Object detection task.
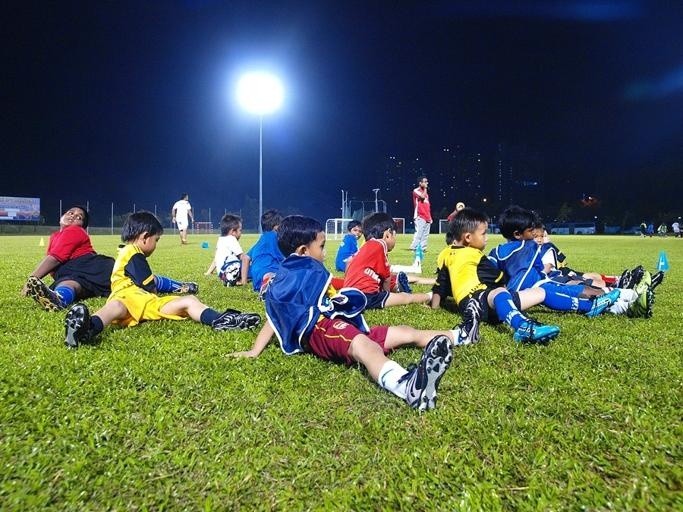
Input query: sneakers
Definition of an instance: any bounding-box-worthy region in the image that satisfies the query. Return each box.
[181,281,199,294]
[629,286,656,319]
[452,298,482,344]
[616,265,663,289]
[64,303,92,347]
[396,270,411,293]
[26,276,64,313]
[413,255,422,273]
[212,309,261,331]
[398,334,453,409]
[513,319,561,344]
[584,288,622,319]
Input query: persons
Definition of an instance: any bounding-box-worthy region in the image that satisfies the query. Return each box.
[448,202,465,221]
[225,215,483,412]
[171,193,195,243]
[204,214,251,287]
[534,214,664,292]
[487,206,653,319]
[335,220,437,284]
[648,221,656,237]
[411,175,433,253]
[421,207,621,346]
[236,209,287,292]
[657,222,668,236]
[544,223,623,283]
[63,211,261,348]
[342,213,434,309]
[432,223,457,274]
[26,206,199,314]
[639,219,647,236]
[672,219,681,237]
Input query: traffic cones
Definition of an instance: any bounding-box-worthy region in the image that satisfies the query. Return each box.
[39,236,45,246]
[656,251,671,272]
